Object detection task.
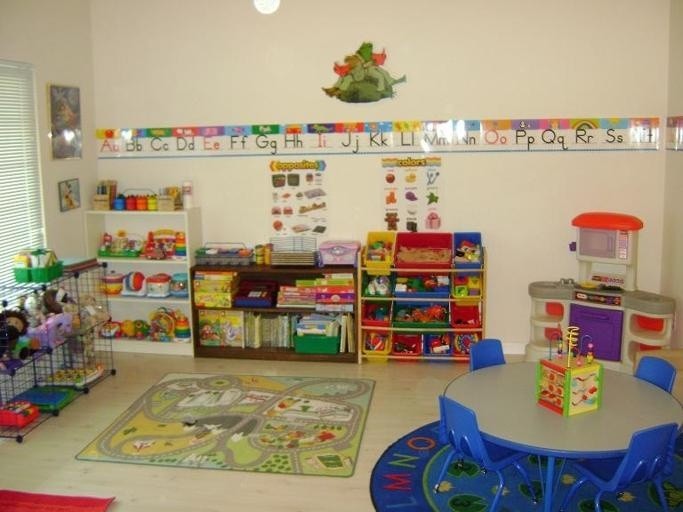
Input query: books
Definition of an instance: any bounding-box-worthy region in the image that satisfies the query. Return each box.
[294,312,340,336]
[243,312,302,349]
[336,312,357,352]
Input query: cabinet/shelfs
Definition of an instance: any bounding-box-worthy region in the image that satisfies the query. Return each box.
[190,263,357,363]
[0,259,117,445]
[83,207,202,357]
[524,279,677,368]
[357,244,488,365]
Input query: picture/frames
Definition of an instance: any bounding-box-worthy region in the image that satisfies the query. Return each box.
[58,177,82,212]
[47,83,84,163]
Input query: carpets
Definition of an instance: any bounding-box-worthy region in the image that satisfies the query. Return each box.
[369,418,682,512]
[74,370,376,478]
[0,489,116,512]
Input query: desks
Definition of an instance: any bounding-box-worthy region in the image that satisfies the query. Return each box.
[443,359,682,512]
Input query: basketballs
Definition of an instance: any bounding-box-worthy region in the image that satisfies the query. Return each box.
[126,271,145,291]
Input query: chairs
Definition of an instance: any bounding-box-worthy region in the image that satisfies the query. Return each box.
[630,354,674,395]
[434,394,537,512]
[560,423,679,512]
[470,338,507,375]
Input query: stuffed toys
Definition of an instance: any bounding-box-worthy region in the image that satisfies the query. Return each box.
[23,290,73,352]
[0,311,39,377]
[40,288,112,329]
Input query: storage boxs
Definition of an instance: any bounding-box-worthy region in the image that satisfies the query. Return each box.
[363,231,397,275]
[392,300,449,336]
[394,276,451,307]
[451,301,483,334]
[361,300,391,335]
[393,232,454,276]
[30,260,64,284]
[10,266,30,284]
[317,241,360,268]
[391,333,422,363]
[454,231,483,277]
[452,277,483,306]
[363,330,392,364]
[452,331,483,365]
[362,271,394,298]
[424,332,453,363]
[292,333,341,357]
